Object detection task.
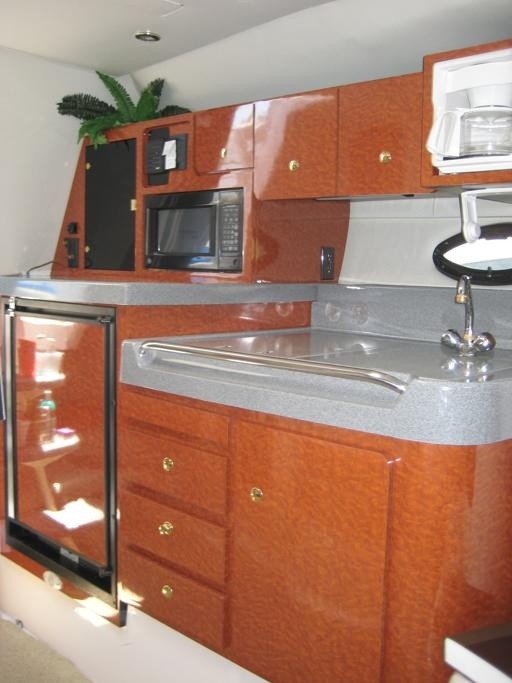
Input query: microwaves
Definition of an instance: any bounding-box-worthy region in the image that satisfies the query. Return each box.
[143,188,245,272]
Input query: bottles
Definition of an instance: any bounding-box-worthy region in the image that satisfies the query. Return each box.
[39,388,57,434]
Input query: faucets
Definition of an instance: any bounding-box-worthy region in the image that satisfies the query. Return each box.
[455,274,474,343]
